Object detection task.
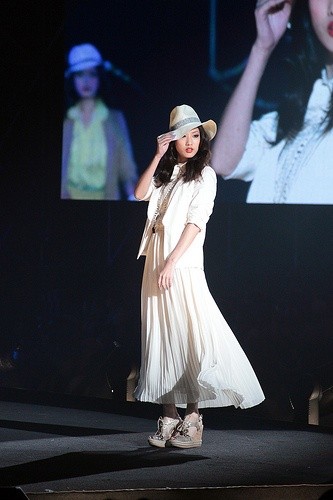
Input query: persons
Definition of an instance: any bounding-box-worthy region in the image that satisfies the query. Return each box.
[130,105,265,448]
[210,0,333,204]
[59,43,139,201]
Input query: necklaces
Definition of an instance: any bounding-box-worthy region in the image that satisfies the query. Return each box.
[153,173,182,220]
[272,124,317,202]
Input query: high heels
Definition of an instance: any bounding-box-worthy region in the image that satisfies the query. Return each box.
[147,417,182,448]
[167,414,204,448]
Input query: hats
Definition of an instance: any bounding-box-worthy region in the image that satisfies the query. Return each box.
[154,104,219,143]
